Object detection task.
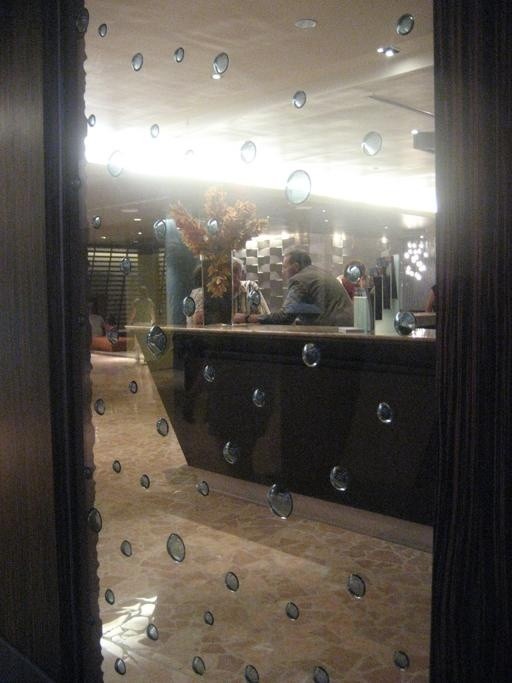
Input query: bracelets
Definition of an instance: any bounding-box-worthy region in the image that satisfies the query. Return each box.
[243,313,251,324]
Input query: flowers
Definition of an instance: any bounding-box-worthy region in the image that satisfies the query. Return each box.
[167,185,270,300]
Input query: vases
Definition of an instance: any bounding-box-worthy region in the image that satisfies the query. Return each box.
[201,248,235,327]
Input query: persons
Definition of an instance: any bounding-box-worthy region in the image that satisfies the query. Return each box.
[233,259,271,313]
[128,285,155,326]
[231,250,357,325]
[425,283,436,315]
[186,265,206,327]
[335,259,369,297]
